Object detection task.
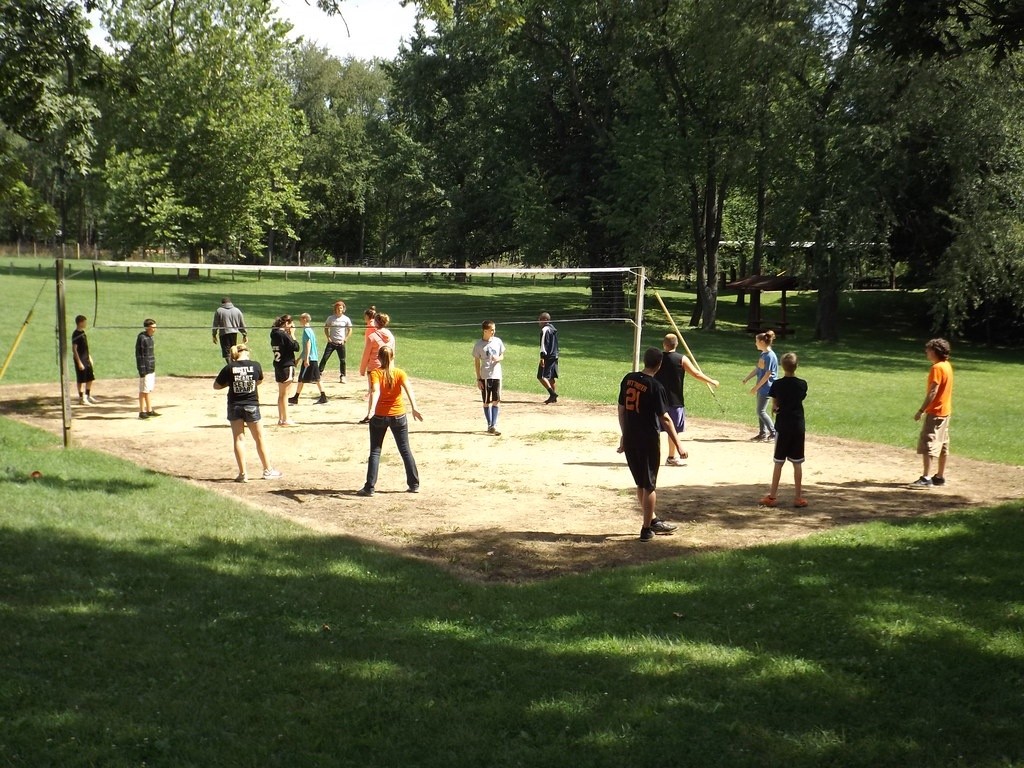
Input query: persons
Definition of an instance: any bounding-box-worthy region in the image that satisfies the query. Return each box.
[537,312,559,404]
[359,313,395,424]
[289,313,327,404]
[212,298,247,364]
[72,315,99,405]
[136,319,162,419]
[355,346,424,497]
[364,306,377,343]
[270,314,300,424]
[742,330,777,443]
[318,301,352,382]
[653,334,719,467]
[909,337,953,486]
[758,352,809,507]
[616,346,688,542]
[214,344,282,482]
[472,320,505,435]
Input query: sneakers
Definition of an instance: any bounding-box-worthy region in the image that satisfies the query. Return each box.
[263,468,283,479]
[907,477,933,489]
[931,473,945,486]
[764,432,777,442]
[758,496,776,506]
[650,519,679,534]
[665,457,688,467]
[794,498,809,507]
[357,488,374,497]
[749,432,766,443]
[640,527,656,541]
[409,483,419,493]
[359,417,371,423]
[235,474,248,483]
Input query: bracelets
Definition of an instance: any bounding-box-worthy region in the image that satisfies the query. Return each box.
[919,409,924,413]
[477,379,481,381]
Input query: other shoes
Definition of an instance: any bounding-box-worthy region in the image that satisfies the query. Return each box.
[79,398,92,406]
[139,412,150,420]
[340,376,347,383]
[147,411,162,416]
[544,393,558,404]
[488,425,502,435]
[288,397,298,404]
[313,400,329,404]
[87,397,100,404]
[278,418,301,427]
